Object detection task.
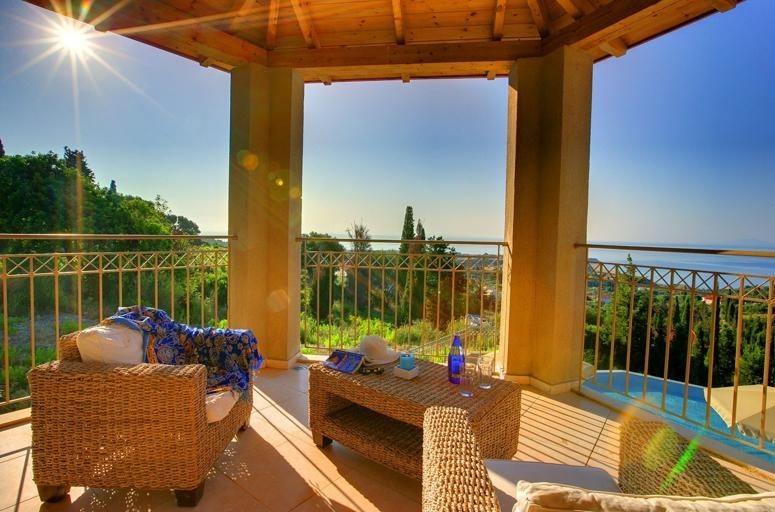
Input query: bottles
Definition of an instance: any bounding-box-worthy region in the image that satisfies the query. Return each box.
[448,331,465,384]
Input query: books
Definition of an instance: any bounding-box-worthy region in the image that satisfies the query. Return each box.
[323,349,376,375]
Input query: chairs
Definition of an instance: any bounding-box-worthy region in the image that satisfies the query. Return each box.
[27,302,258,505]
[421,393,775,511]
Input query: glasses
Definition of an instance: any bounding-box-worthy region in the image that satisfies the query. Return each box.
[358,366,384,375]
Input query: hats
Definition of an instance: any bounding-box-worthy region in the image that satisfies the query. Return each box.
[349,335,401,366]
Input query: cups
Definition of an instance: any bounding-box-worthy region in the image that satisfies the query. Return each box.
[459,356,493,398]
